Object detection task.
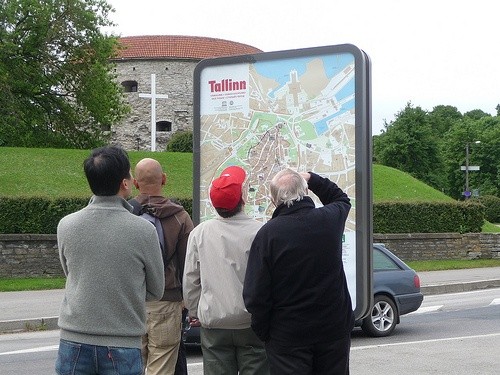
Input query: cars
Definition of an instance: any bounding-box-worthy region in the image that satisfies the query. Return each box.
[182,243,424,347]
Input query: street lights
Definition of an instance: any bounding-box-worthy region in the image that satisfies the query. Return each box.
[466,140,481,199]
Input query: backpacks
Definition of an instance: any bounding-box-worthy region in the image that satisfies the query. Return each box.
[138,212,166,264]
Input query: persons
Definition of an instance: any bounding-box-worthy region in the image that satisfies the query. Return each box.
[128,158,196,375]
[55,147,165,375]
[243,168,356,375]
[182,166,268,375]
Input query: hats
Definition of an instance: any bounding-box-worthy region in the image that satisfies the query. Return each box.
[210,166,246,211]
